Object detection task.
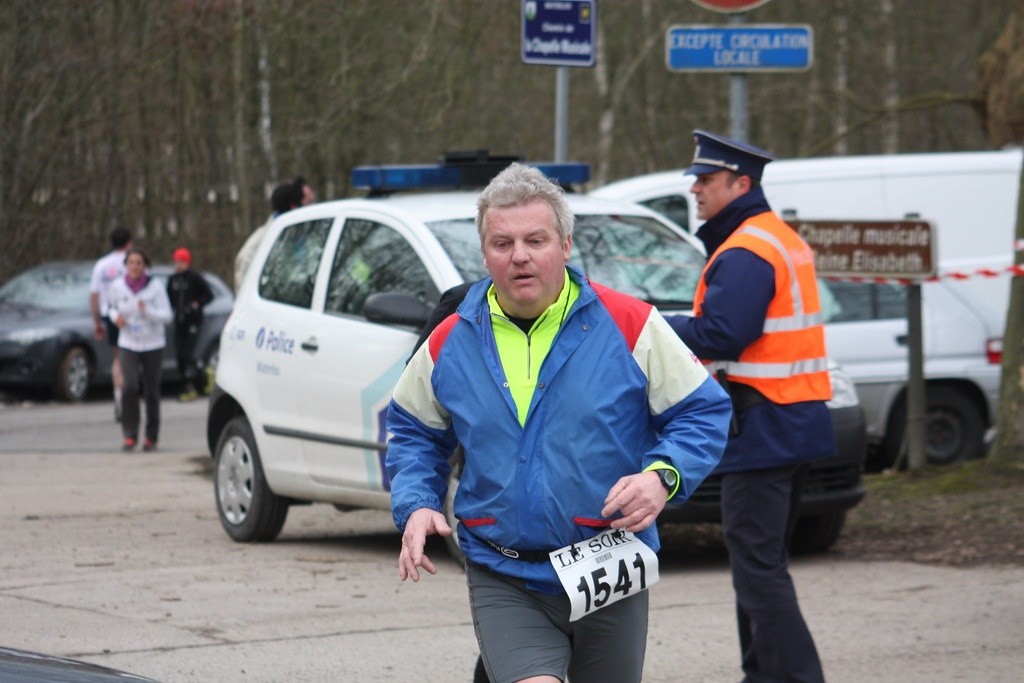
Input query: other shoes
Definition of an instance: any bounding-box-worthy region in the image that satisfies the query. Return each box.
[203,367,214,394]
[123,437,137,450]
[179,389,198,401]
[143,438,153,450]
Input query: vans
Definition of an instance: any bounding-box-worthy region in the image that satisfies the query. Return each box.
[581,147,1024,471]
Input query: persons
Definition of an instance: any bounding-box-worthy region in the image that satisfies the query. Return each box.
[380,130,837,683]
[90,224,215,452]
[271,176,316,217]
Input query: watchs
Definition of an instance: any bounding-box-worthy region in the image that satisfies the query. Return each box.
[654,469,677,495]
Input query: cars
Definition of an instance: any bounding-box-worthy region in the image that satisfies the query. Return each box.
[204,147,868,559]
[1,255,234,402]
[819,272,996,466]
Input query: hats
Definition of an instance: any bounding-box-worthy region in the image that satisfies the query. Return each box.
[683,128,774,181]
[173,248,192,264]
